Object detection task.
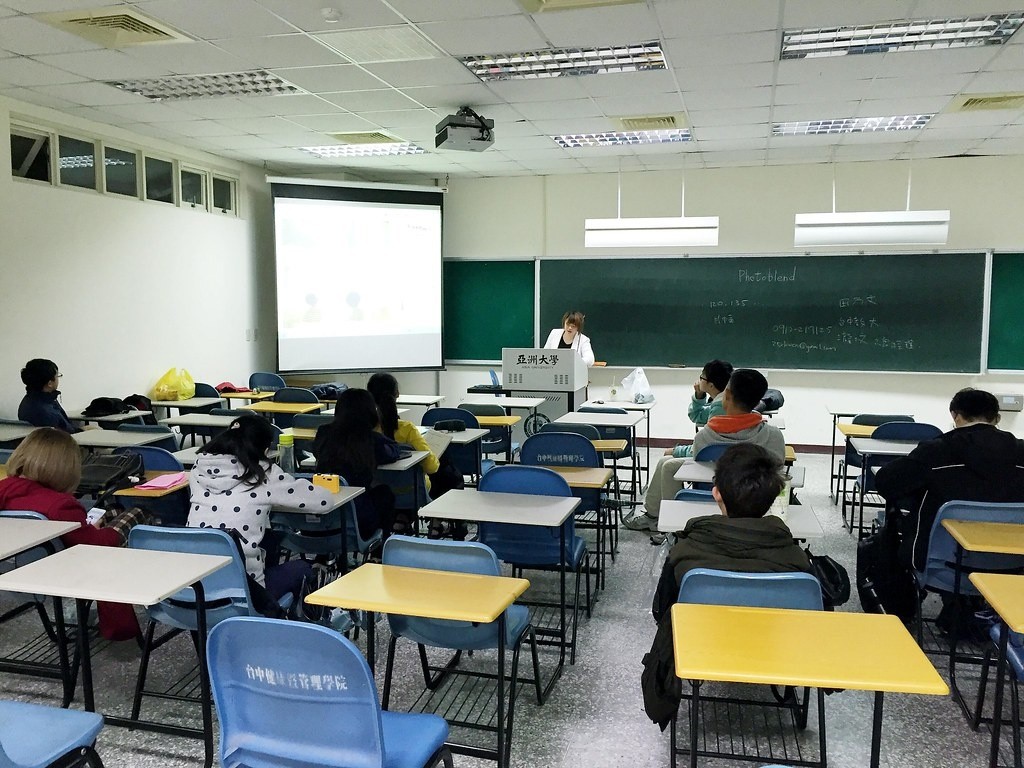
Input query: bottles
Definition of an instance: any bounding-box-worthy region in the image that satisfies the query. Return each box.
[277,434,294,477]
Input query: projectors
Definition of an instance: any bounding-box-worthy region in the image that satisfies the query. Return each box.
[434,126,495,152]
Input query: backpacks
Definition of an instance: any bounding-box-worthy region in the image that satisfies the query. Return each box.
[853,511,923,624]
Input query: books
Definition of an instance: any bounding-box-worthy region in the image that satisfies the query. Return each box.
[424,429,453,459]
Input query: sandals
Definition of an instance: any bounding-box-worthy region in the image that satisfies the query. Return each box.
[427,524,450,540]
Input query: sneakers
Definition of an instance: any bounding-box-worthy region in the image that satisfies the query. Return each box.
[625,510,658,531]
[650,533,668,545]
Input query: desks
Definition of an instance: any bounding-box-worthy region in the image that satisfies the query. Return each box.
[0,392,1024,768]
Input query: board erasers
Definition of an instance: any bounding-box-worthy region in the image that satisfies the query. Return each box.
[593,361,607,367]
[669,364,685,368]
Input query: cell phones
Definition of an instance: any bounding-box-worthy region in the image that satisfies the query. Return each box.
[86,507,106,524]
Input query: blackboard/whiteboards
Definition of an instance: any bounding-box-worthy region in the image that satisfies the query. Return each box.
[538,248,989,375]
[986,250,1024,374]
[442,256,537,364]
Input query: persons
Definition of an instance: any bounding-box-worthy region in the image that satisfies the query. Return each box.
[693,369,785,467]
[544,312,594,367]
[641,442,811,732]
[17,358,113,459]
[367,372,452,537]
[0,427,157,639]
[877,388,1024,647]
[313,389,400,563]
[624,359,734,544]
[186,415,333,629]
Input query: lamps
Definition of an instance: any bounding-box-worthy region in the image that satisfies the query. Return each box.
[583,152,720,248]
[792,139,951,247]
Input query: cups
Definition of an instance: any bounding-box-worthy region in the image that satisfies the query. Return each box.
[771,474,793,521]
[608,386,618,402]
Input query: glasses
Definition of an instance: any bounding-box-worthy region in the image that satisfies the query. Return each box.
[53,373,63,379]
[699,374,708,382]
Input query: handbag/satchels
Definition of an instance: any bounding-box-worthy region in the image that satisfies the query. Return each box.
[310,381,348,399]
[148,367,195,401]
[621,368,655,404]
[285,553,339,624]
[84,397,129,416]
[76,450,145,491]
[761,390,784,410]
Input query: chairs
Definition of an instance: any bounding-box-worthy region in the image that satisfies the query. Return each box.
[117,423,180,453]
[0,698,106,768]
[457,403,521,465]
[380,533,543,768]
[692,442,734,491]
[0,509,100,644]
[376,443,435,510]
[249,372,287,424]
[669,566,825,768]
[989,622,1024,768]
[210,408,257,417]
[476,464,591,667]
[673,488,716,501]
[178,383,223,450]
[834,413,916,529]
[203,616,455,768]
[293,413,335,473]
[0,448,15,465]
[127,404,145,425]
[0,418,34,427]
[906,499,1024,658]
[850,420,944,542]
[421,407,495,488]
[539,422,627,554]
[127,524,294,733]
[577,406,643,510]
[520,432,615,574]
[110,446,191,524]
[273,387,322,469]
